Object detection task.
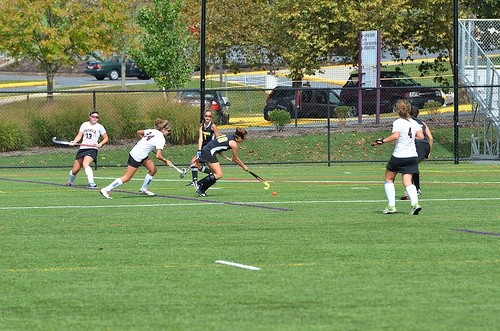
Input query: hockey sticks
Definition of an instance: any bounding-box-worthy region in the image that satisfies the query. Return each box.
[52,137,102,148]
[151,149,186,174]
[180,156,199,179]
[219,153,270,190]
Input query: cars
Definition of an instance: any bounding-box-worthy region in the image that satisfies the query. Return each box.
[83,54,151,80]
[177,88,231,125]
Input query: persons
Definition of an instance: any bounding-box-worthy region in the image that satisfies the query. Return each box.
[303,82,311,86]
[99,119,171,199]
[401,107,433,200]
[187,110,220,186]
[371,100,425,215]
[66,112,108,187]
[192,127,250,197]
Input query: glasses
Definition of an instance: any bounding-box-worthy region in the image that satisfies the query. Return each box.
[91,116,99,119]
[166,129,171,132]
[204,115,212,118]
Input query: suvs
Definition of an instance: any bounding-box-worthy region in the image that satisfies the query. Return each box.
[263,86,341,121]
[340,71,445,117]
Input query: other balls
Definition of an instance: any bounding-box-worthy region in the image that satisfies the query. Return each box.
[272,192,278,196]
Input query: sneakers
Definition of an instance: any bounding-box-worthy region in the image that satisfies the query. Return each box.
[139,188,155,196]
[383,206,397,214]
[100,187,113,199]
[193,180,199,191]
[409,203,422,216]
[88,183,97,187]
[401,189,421,200]
[196,190,206,197]
[65,182,72,186]
